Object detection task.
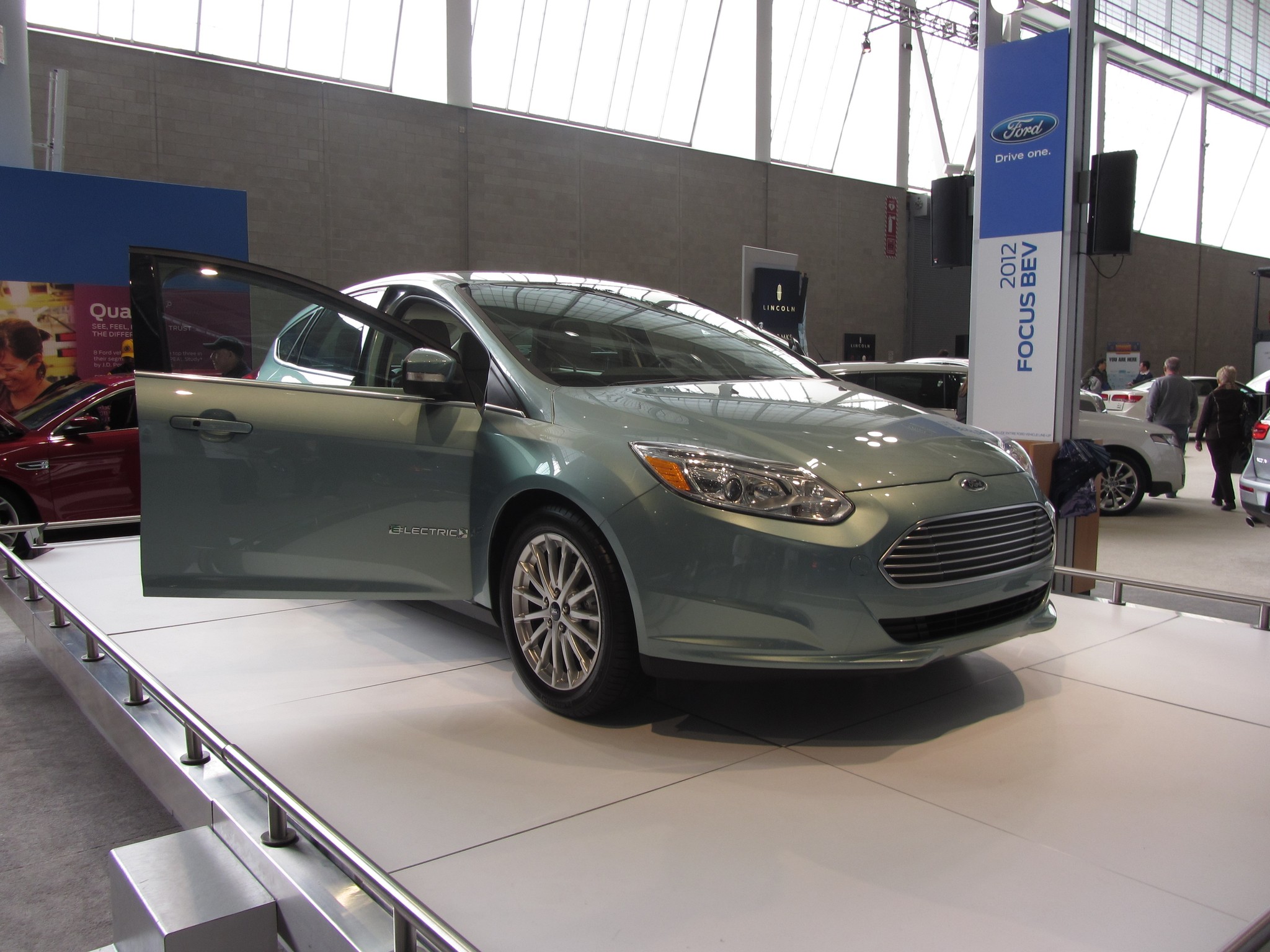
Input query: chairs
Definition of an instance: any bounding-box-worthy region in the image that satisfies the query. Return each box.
[537,319,595,371]
[109,399,138,429]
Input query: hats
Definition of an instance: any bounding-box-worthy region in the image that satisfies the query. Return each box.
[121,338,134,359]
[203,336,245,357]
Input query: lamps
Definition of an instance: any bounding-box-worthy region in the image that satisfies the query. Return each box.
[968,12,978,22]
[861,34,871,54]
[902,42,912,51]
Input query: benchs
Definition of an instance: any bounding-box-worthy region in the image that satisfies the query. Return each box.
[388,320,490,406]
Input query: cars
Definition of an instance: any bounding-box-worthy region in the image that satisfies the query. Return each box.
[2,370,223,557]
[129,234,1056,715]
[819,357,1269,529]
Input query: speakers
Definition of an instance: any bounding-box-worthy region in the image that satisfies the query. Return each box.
[1086,150,1139,256]
[931,174,975,270]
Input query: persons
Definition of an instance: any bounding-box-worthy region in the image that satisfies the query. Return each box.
[1194,365,1245,510]
[1080,359,1112,395]
[0,318,64,412]
[104,337,138,430]
[937,349,949,357]
[1125,361,1153,386]
[201,336,252,378]
[64,375,101,434]
[1146,357,1199,497]
